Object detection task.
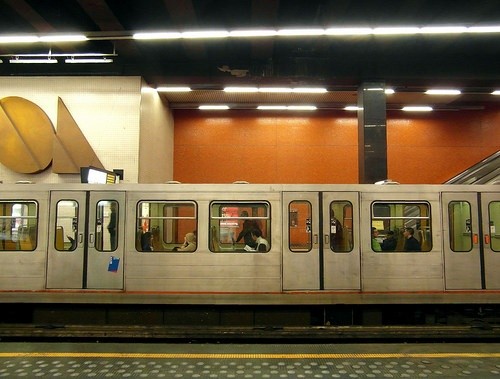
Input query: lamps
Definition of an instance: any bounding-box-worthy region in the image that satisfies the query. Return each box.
[0,48,117,64]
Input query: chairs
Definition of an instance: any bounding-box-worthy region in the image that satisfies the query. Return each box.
[395,228,428,251]
[137,225,164,251]
[211,225,220,251]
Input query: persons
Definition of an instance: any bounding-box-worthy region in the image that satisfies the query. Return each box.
[235,210,262,251]
[330,208,343,253]
[371,227,383,252]
[248,228,270,253]
[140,230,154,252]
[106,201,117,251]
[379,230,398,252]
[403,226,421,252]
[177,232,197,253]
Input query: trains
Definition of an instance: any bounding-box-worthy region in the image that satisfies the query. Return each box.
[0,178,500,343]
